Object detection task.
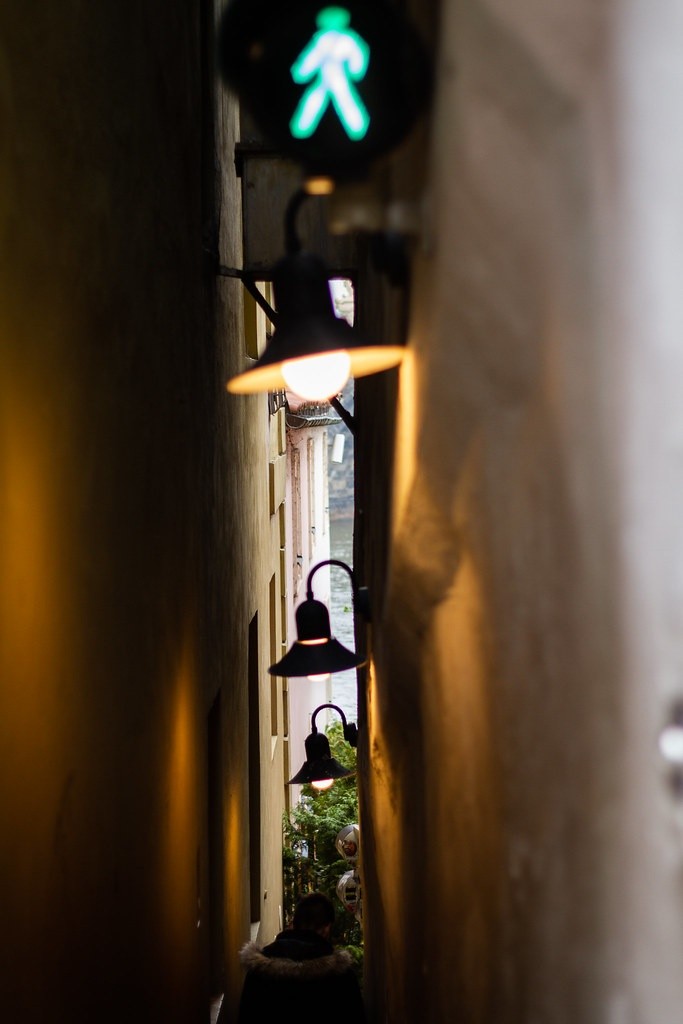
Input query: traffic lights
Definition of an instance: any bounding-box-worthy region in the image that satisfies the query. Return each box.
[217,0,439,171]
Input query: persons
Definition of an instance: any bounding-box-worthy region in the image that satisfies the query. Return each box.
[237,892,367,1024]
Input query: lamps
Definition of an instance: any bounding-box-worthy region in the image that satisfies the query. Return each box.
[267,560,373,678]
[287,703,358,789]
[225,160,405,404]
